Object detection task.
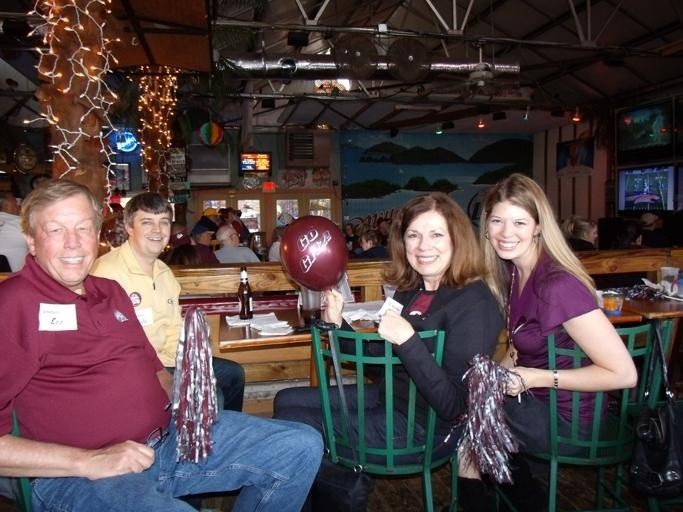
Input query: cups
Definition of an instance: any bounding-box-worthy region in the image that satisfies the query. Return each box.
[661,266,679,298]
[302,286,321,327]
[602,293,624,315]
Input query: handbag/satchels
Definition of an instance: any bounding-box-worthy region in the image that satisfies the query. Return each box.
[626,318,682,499]
[309,460,369,512]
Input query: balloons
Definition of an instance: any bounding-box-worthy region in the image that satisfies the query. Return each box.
[279,217,347,292]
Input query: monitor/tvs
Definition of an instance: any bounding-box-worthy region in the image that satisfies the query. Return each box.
[615,160,683,214]
[239,152,272,176]
[615,95,679,163]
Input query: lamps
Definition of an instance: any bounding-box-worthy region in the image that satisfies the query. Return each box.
[433,106,581,135]
[389,108,506,138]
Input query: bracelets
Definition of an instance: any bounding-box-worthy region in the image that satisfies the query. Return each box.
[552,368,559,392]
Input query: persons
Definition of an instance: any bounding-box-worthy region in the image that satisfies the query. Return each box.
[456,173,640,510]
[616,217,649,252]
[560,212,598,250]
[270,188,509,511]
[169,204,391,270]
[639,212,670,248]
[85,193,244,416]
[0,175,325,511]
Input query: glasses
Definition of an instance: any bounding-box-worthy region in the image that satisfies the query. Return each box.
[146,427,170,452]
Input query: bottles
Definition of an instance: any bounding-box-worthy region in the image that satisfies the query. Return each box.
[238,265,254,319]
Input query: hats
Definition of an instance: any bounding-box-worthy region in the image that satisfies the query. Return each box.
[191,225,214,234]
[639,212,659,226]
[203,207,221,217]
